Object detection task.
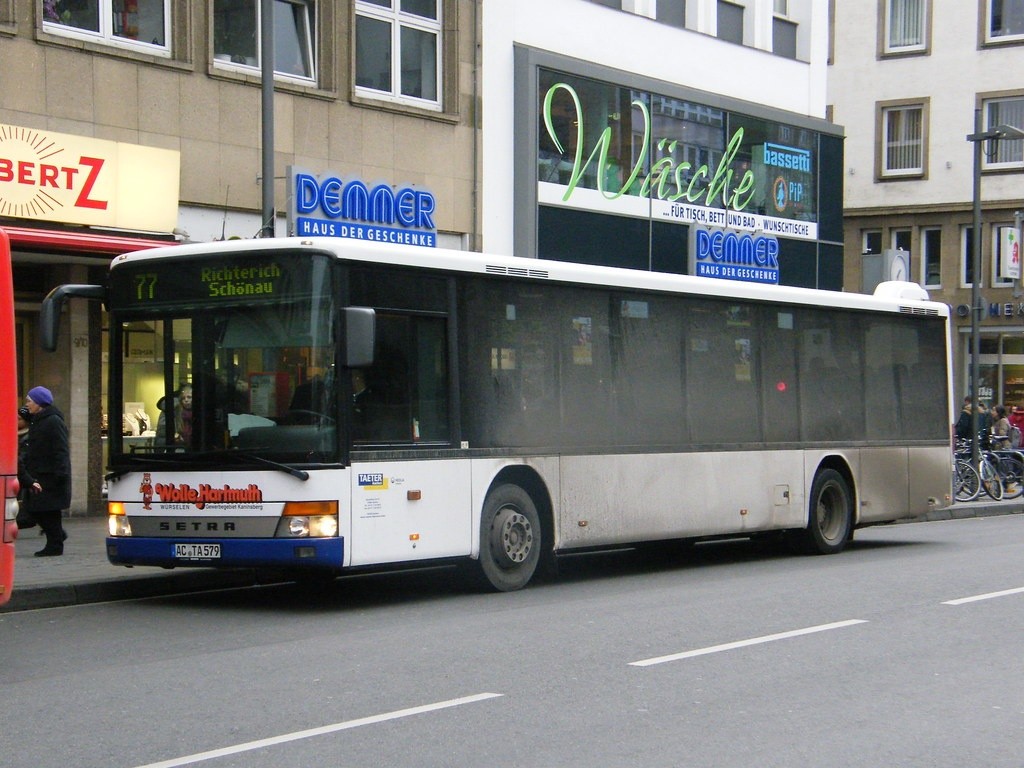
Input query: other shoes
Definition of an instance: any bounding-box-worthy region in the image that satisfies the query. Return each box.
[34,532,69,556]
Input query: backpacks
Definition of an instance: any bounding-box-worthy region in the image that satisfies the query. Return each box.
[1004,423,1022,449]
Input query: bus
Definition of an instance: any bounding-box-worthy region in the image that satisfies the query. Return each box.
[40,237,953,592]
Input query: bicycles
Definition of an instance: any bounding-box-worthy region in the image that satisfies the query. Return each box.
[953,435,1024,502]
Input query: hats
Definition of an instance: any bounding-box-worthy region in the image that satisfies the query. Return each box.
[28,386,53,407]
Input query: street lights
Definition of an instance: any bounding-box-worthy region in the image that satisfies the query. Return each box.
[966,125,1024,501]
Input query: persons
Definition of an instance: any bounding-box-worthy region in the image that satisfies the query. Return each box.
[16,406,33,529]
[953,390,1024,493]
[152,372,231,456]
[25,386,73,558]
